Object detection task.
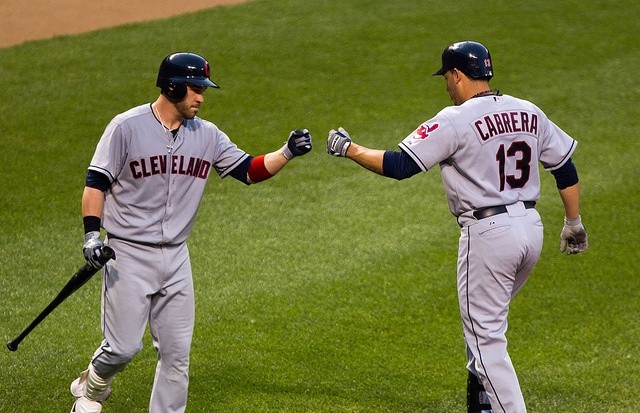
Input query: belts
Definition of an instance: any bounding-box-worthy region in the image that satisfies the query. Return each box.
[107,231,122,242]
[457,200,537,229]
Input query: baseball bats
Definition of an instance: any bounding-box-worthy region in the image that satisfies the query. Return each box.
[6,246,114,352]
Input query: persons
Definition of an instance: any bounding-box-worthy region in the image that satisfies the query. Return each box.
[68,52,314,413]
[325,41,589,413]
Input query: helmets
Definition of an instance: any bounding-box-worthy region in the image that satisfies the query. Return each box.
[156,52,220,102]
[433,40,494,82]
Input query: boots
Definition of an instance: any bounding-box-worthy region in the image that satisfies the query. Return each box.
[69,359,114,413]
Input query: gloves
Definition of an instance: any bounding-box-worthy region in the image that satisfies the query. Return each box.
[326,126,351,157]
[83,229,104,270]
[559,216,588,256]
[282,129,312,160]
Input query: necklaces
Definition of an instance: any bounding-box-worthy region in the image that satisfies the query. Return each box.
[469,89,500,98]
[152,103,184,154]
